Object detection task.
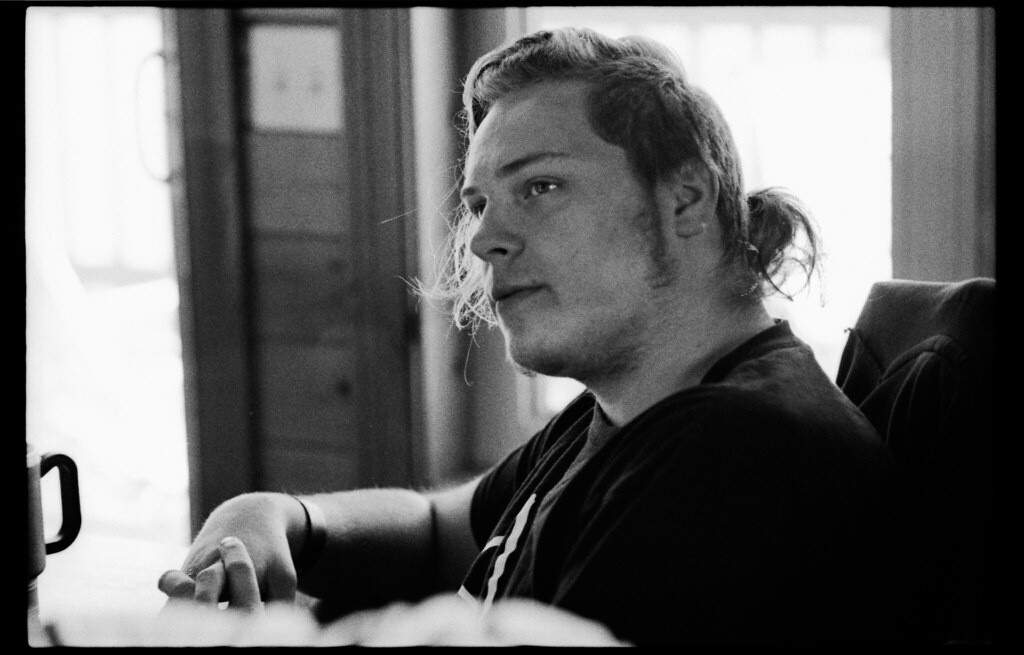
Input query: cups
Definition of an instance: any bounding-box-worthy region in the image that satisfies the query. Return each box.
[26,443,81,584]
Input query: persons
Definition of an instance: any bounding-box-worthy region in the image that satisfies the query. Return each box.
[160,29,906,646]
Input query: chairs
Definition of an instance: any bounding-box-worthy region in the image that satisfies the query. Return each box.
[838,272,996,653]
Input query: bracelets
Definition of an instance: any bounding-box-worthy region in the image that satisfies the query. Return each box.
[287,491,329,575]
